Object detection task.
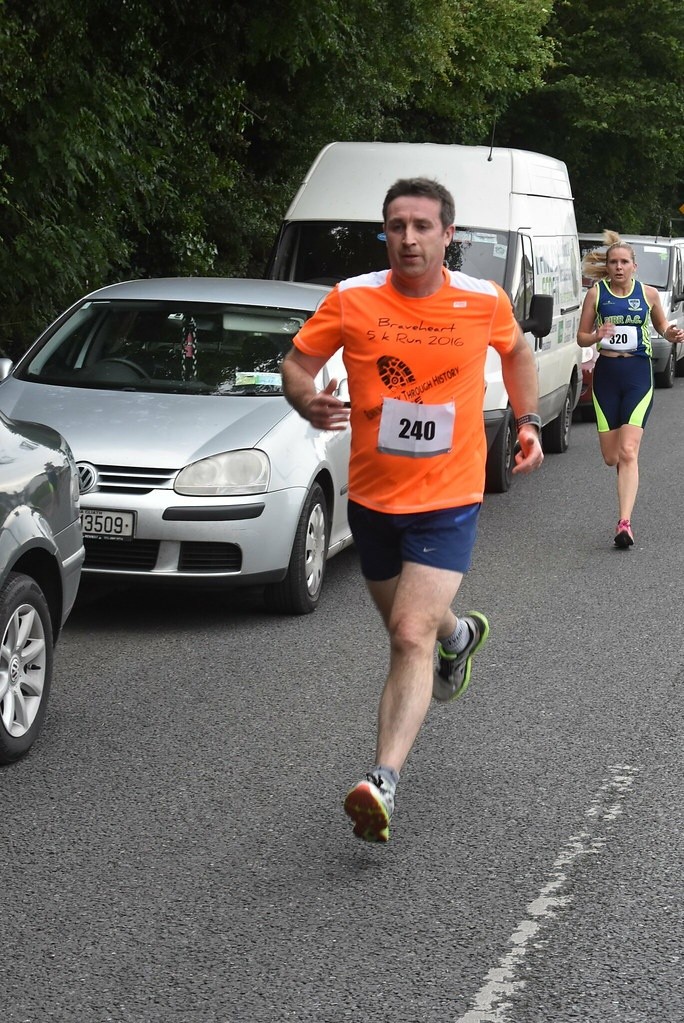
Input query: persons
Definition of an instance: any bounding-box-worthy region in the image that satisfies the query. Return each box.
[277,177,546,842]
[577,230,683,548]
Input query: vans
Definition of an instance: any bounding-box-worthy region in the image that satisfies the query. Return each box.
[270,114,583,493]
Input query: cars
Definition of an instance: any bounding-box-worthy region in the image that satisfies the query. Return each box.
[0,407,86,759]
[579,233,684,388]
[577,330,598,424]
[2,277,353,611]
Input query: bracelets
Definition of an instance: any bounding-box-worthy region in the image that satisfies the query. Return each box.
[515,413,541,435]
[662,330,672,343]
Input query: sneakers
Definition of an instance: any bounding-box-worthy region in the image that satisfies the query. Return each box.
[614,520,634,548]
[342,772,393,843]
[432,610,490,703]
